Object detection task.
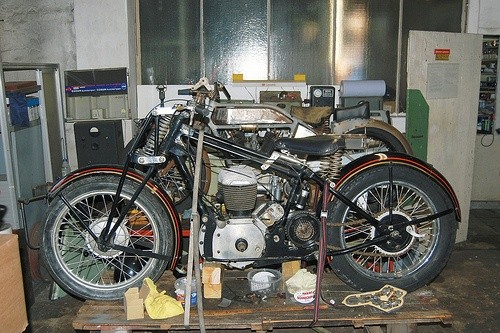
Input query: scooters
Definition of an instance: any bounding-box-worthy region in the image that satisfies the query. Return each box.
[38,98,462,302]
